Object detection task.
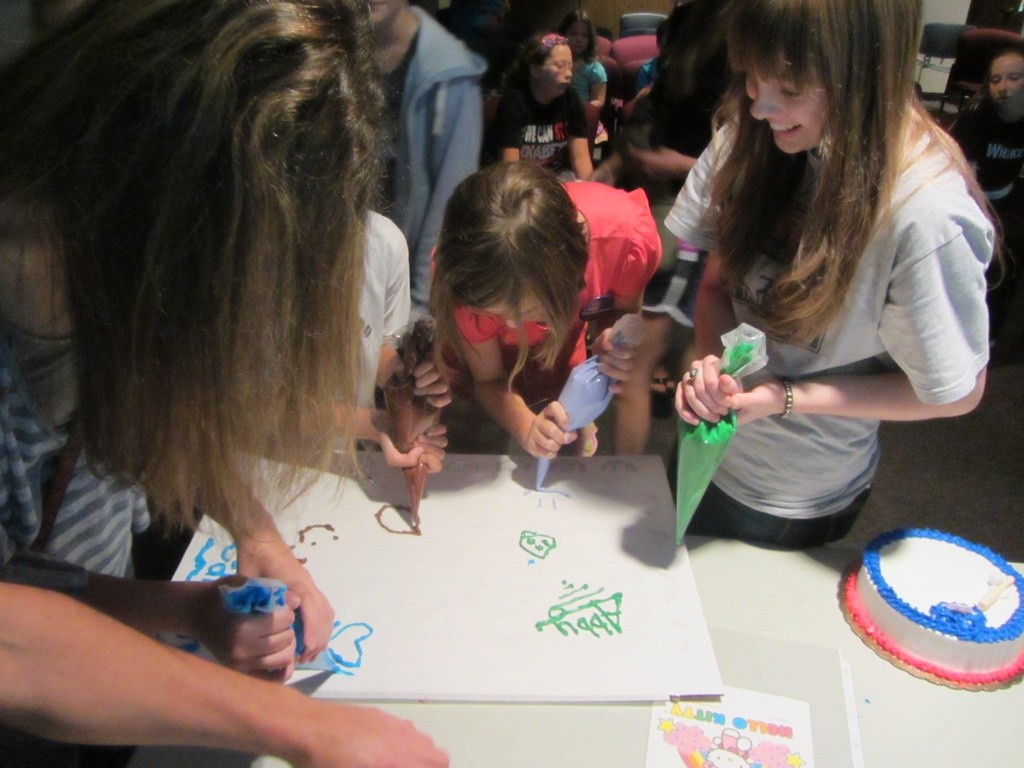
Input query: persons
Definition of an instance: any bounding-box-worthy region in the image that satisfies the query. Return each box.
[0,0,382,768]
[0,581,448,768]
[430,163,664,460]
[952,47,1024,351]
[368,1,487,326]
[665,0,997,548]
[321,207,452,475]
[436,0,738,195]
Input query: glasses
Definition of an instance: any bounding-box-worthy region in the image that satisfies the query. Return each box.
[578,295,613,324]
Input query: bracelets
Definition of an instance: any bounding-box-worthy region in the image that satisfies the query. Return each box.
[781,380,792,420]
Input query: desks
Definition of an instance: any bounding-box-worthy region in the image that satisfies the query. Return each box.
[124,533,1024,768]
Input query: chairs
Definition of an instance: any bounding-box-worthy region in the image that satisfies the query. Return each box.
[588,12,668,161]
[910,23,1024,130]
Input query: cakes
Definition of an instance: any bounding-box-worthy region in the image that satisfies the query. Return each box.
[846,527,1024,684]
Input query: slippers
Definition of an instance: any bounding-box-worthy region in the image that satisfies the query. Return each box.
[651,374,676,399]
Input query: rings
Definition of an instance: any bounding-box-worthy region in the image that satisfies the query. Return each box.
[689,368,703,380]
